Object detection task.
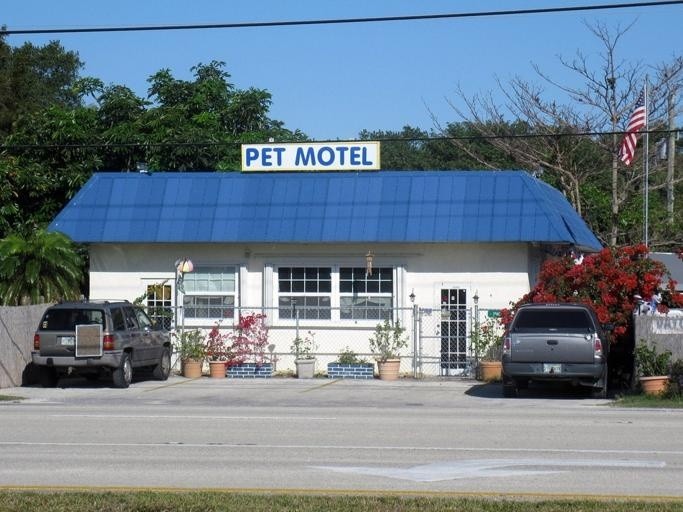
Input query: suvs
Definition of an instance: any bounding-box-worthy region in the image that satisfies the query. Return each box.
[501,301,615,398]
[30,299,175,389]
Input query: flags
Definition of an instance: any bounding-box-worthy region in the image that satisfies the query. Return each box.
[618,83,647,165]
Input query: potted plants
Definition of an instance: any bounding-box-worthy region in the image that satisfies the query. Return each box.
[469,318,504,381]
[292,314,411,380]
[179,330,229,379]
[632,336,673,398]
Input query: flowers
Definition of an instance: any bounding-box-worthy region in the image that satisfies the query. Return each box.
[209,312,274,371]
